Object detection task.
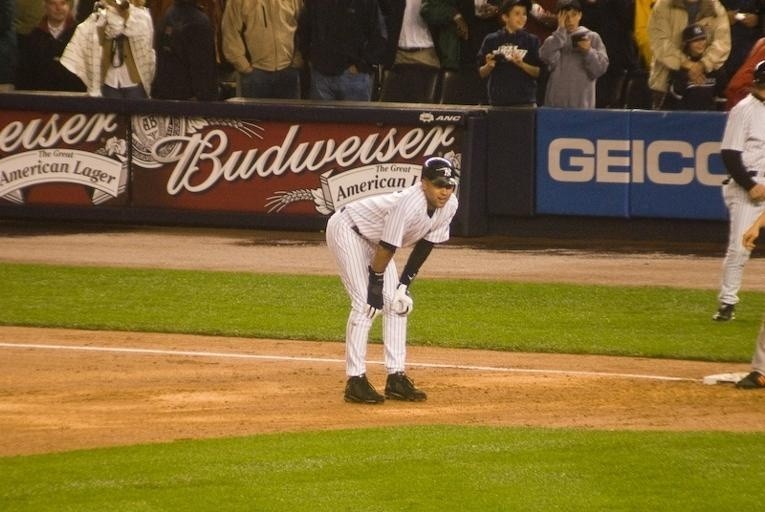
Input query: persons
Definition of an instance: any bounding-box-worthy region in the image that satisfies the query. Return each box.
[1,0,765,110]
[734,212,765,389]
[711,60,765,321]
[325,157,458,404]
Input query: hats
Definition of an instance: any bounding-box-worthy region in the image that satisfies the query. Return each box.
[682,24,707,43]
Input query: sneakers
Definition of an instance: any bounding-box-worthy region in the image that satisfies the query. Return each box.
[734,371,765,389]
[343,374,385,404]
[711,301,736,322]
[383,370,427,402]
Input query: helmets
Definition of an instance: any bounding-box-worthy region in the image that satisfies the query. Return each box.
[752,59,765,83]
[420,157,461,186]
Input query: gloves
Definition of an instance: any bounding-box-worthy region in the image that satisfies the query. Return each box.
[364,280,385,319]
[390,276,413,316]
